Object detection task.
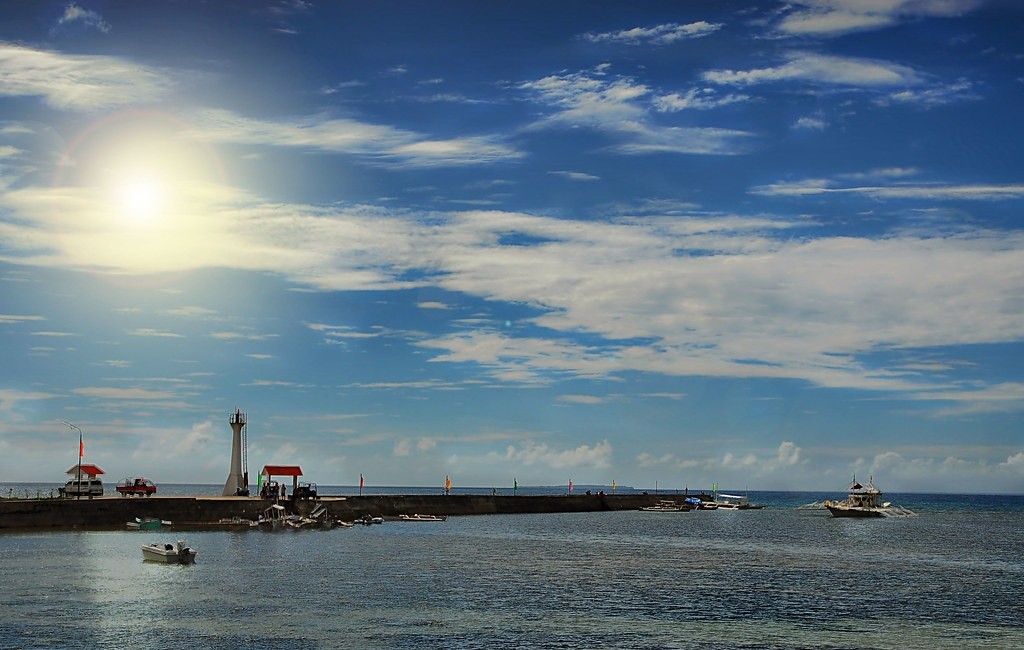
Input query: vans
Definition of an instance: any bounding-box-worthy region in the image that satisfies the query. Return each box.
[65,479,103,496]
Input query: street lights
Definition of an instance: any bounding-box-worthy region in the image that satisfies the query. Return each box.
[61,421,82,498]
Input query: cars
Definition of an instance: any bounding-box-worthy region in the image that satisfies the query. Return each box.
[718,507,738,511]
[219,516,253,524]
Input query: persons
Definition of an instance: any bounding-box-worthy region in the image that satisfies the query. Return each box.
[281,484,286,500]
[266,483,271,492]
[274,483,279,494]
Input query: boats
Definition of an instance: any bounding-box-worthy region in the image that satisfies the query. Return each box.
[141,540,197,562]
[125,517,173,526]
[639,500,690,512]
[797,501,827,510]
[718,495,766,509]
[695,503,718,510]
[354,518,383,523]
[825,472,918,518]
[399,514,442,520]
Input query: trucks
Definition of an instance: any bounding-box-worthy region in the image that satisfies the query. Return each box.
[116,477,156,497]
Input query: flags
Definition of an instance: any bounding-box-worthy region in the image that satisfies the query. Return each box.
[570,481,573,491]
[613,482,616,490]
[259,474,263,490]
[361,477,364,488]
[447,479,451,490]
[514,481,518,490]
